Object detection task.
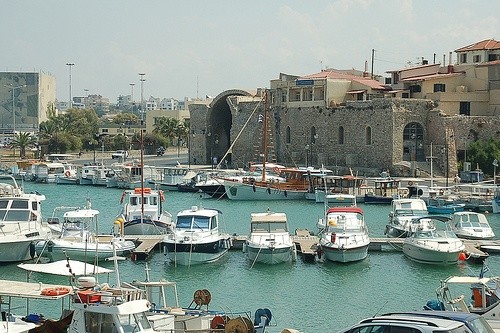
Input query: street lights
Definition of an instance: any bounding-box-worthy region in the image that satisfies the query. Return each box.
[65,63,75,109]
[137,72,147,125]
[129,83,136,126]
[4,84,27,162]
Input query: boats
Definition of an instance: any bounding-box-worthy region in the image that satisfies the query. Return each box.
[451,212,495,240]
[243,207,297,266]
[0,88,500,333]
[159,202,233,266]
[318,206,371,265]
[384,189,437,239]
[403,215,465,267]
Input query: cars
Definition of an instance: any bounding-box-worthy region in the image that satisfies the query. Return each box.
[111,150,128,159]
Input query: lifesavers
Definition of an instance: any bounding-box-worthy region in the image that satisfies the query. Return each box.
[160,191,165,201]
[120,193,125,204]
[41,286,70,295]
[408,181,414,186]
[65,171,71,176]
